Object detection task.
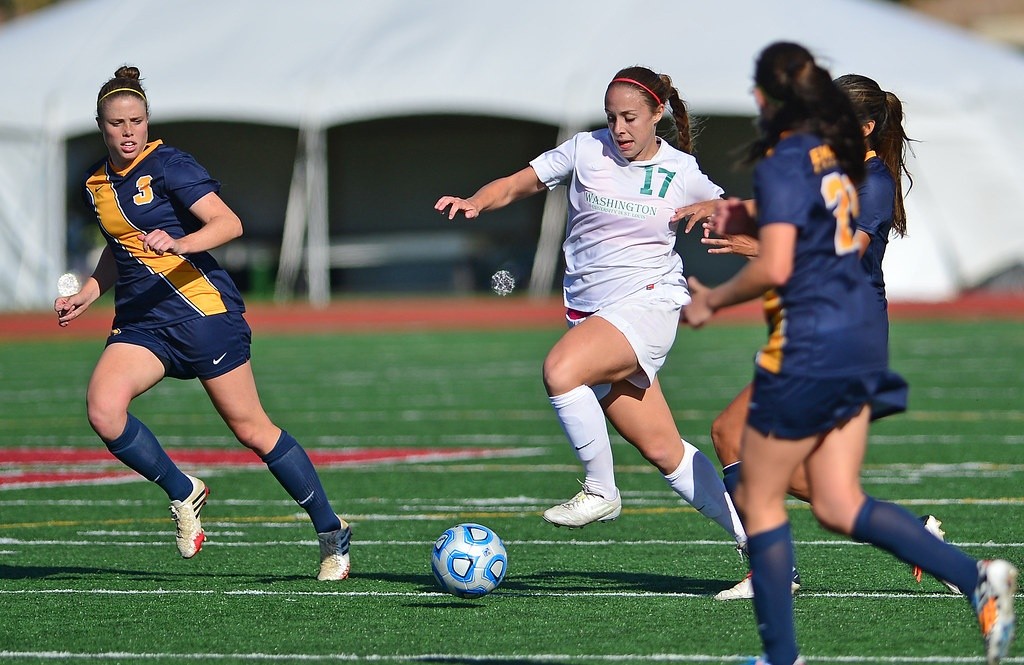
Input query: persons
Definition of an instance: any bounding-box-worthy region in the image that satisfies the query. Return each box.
[53,66,352,582]
[672,39,1018,664]
[434,66,800,601]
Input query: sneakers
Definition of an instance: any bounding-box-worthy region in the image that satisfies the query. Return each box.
[542,485,622,530]
[714,569,802,600]
[969,558,1018,664]
[316,512,353,581]
[168,473,211,558]
[915,514,963,595]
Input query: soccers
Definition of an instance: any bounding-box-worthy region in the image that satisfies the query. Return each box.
[431,523,508,599]
